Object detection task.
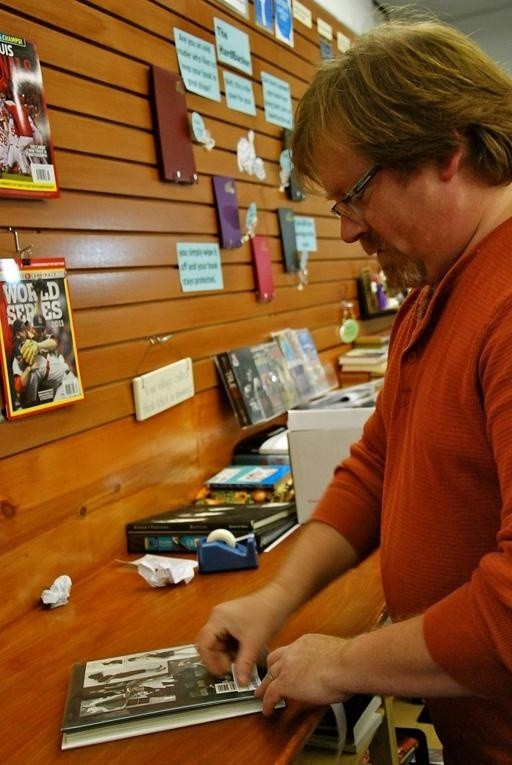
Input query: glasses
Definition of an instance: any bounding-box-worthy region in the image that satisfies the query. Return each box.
[330,164,381,224]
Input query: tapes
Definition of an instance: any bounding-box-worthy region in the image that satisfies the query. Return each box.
[206,528,255,548]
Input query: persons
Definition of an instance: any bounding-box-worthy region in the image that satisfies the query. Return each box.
[194,22,512,765]
[0,93,47,175]
[11,315,81,412]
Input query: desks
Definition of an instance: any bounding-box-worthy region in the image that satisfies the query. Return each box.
[1,372,398,765]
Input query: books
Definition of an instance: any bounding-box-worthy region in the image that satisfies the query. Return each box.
[217,328,330,426]
[60,639,286,751]
[306,694,382,754]
[337,336,391,388]
[397,738,419,765]
[124,424,299,556]
[0,256,86,422]
[0,30,61,201]
